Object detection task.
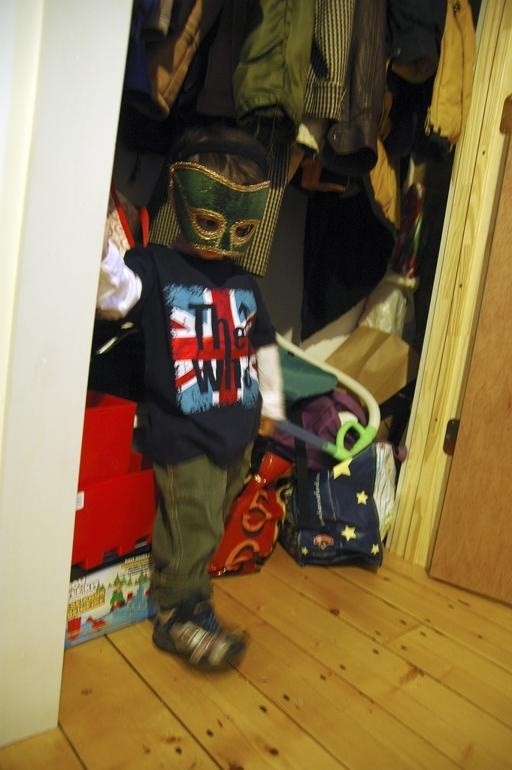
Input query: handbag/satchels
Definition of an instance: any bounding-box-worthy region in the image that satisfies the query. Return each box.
[205,325,419,580]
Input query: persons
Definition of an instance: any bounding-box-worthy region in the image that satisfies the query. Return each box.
[97,126,285,671]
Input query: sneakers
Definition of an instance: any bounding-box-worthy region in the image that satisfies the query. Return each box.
[152,601,247,672]
